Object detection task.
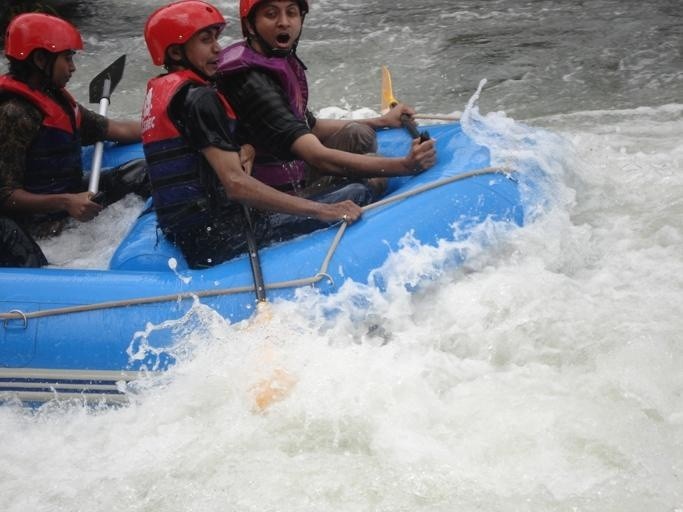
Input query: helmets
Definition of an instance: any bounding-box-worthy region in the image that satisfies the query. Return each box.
[239,0,309,37]
[5,12,84,62]
[144,1,226,67]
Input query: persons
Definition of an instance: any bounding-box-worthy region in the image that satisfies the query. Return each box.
[0,13,150,268]
[137,0,376,269]
[213,0,439,203]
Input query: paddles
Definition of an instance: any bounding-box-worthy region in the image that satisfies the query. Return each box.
[89,54,124,204]
[381,66,429,145]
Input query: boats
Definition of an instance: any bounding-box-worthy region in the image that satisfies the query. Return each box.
[0,119,552,414]
